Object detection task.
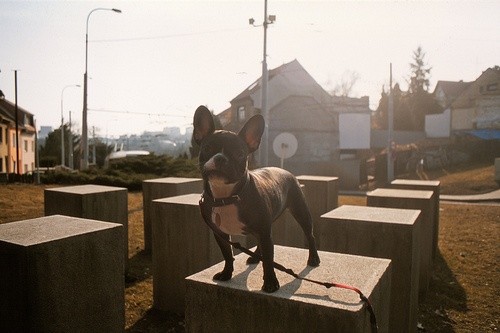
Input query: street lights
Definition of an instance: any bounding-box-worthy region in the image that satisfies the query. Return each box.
[248,0,277,170]
[80,7,122,172]
[60,84,81,166]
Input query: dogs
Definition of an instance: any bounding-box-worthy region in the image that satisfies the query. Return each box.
[191,105,321,293]
